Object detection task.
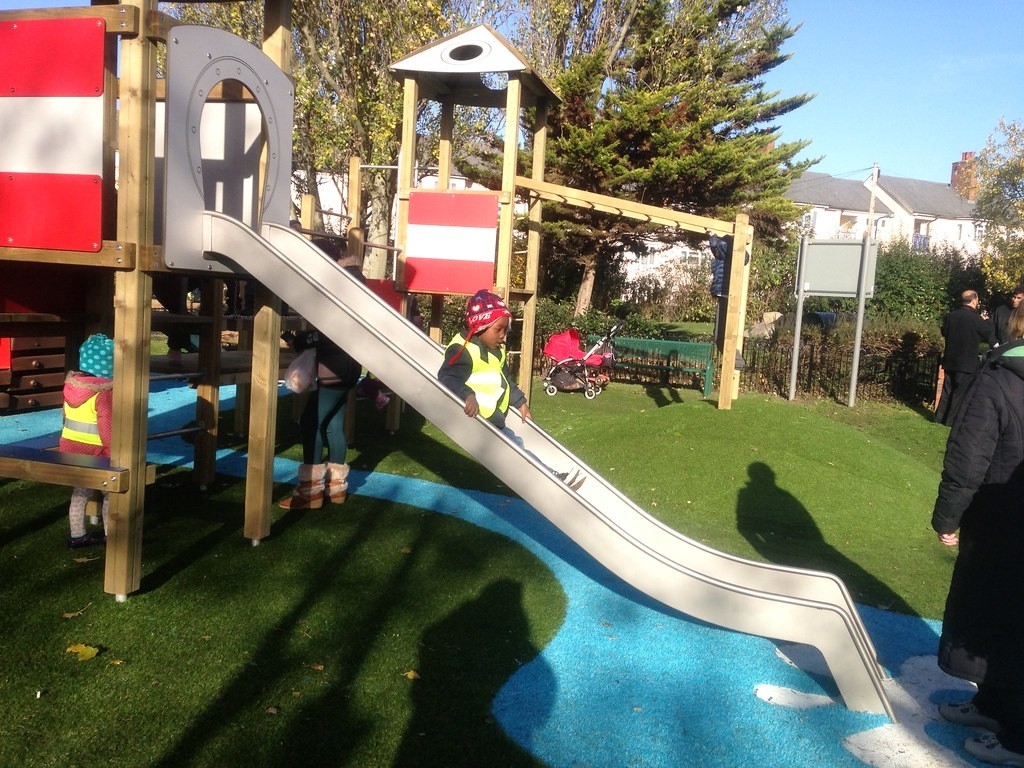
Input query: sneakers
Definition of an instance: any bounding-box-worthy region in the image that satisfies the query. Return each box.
[965,736,1024,767]
[938,702,998,731]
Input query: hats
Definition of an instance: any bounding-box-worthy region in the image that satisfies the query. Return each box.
[466,290,512,335]
[79,333,114,379]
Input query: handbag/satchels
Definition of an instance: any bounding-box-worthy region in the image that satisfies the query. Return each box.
[285,348,317,392]
[317,354,350,385]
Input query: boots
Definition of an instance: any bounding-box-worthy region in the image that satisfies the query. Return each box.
[325,462,350,503]
[280,464,326,509]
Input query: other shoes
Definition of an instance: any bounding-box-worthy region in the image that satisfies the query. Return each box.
[70,534,97,547]
[570,473,588,487]
[553,467,580,485]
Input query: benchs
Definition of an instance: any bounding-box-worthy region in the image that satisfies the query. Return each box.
[585,335,714,398]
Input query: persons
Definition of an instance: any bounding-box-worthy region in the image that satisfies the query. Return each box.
[277,238,367,510]
[436,288,587,491]
[931,298,1024,768]
[57,333,114,546]
[988,285,1024,348]
[932,289,999,428]
[149,268,192,361]
[408,295,423,332]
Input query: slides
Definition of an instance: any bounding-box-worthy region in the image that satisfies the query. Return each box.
[204,210,894,721]
